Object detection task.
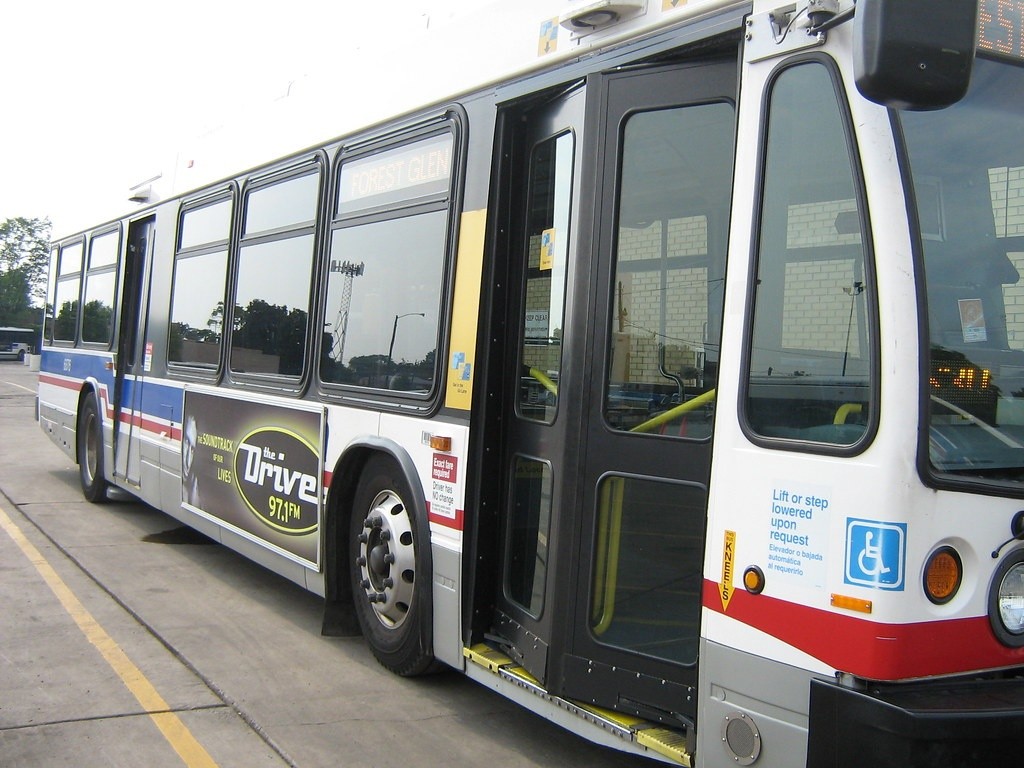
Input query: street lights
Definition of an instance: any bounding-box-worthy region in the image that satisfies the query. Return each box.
[841,281,866,376]
[384,312,426,390]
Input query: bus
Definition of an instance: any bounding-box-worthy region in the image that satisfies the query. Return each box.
[34,0,1024,767]
[0,326,35,361]
